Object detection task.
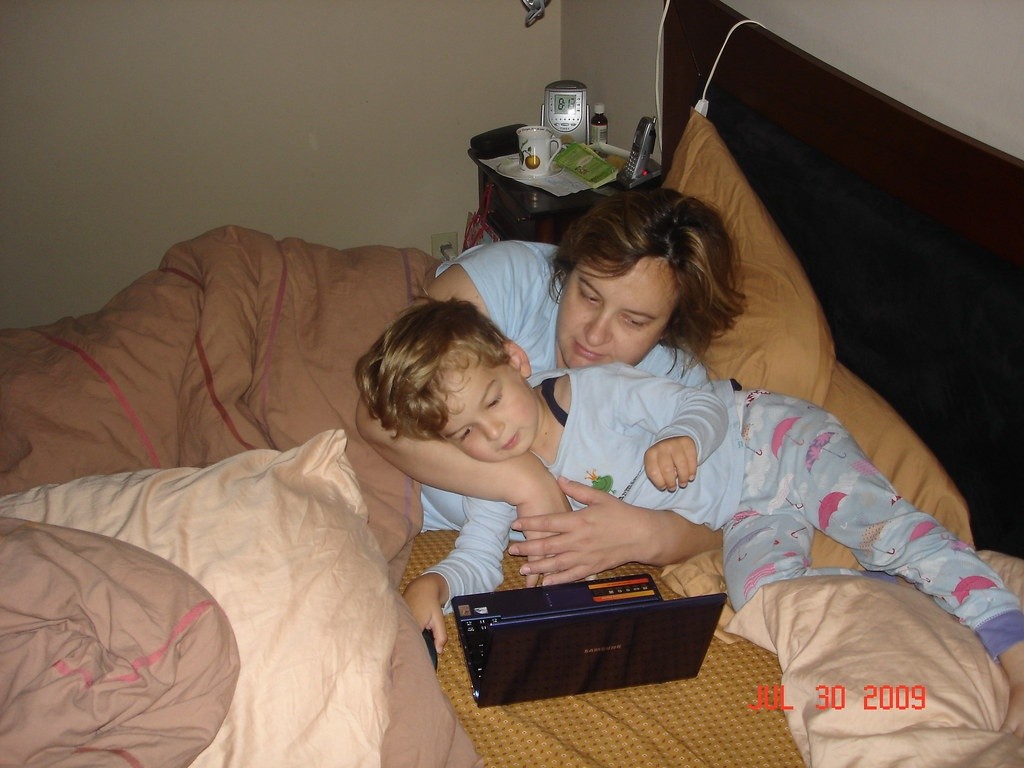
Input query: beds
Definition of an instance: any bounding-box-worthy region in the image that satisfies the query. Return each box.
[0,1,1024,768]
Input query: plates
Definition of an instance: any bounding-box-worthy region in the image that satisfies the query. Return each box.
[498,158,565,179]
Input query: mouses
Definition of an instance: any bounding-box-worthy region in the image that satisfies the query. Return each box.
[422,629,437,672]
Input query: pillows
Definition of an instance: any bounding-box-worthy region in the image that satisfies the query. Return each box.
[728,548,1024,768]
[654,105,836,403]
[654,364,974,646]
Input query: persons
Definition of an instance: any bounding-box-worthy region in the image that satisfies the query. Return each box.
[354,297,1024,740]
[1,179,744,590]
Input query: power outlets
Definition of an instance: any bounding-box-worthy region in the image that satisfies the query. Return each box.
[431,232,458,260]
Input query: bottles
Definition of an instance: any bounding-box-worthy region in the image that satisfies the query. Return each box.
[590,103,608,144]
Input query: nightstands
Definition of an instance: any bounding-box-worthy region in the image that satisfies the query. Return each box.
[466,146,607,244]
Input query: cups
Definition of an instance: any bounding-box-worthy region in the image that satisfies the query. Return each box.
[516,125,562,173]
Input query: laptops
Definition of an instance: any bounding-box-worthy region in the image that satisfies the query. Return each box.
[451,572,727,707]
